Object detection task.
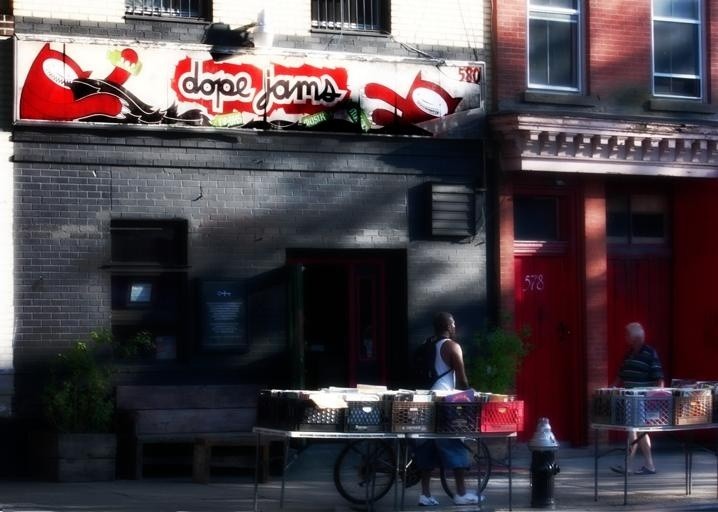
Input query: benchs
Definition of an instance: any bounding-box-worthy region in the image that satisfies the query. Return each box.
[113,381,290,483]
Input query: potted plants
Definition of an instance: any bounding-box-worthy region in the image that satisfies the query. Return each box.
[458,309,536,464]
[35,325,160,482]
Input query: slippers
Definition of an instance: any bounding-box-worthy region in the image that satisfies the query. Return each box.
[635,466,656,474]
[612,464,631,474]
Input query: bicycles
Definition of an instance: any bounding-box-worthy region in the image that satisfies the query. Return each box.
[332,434,492,503]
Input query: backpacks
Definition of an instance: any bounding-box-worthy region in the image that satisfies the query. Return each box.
[406,335,452,390]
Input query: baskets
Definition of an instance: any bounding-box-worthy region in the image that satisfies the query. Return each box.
[256,396,525,432]
[591,395,712,427]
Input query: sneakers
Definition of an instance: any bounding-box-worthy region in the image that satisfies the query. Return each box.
[419,495,439,505]
[455,494,484,505]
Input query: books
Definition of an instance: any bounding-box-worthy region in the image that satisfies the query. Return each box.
[595,378,718,426]
[264,384,517,431]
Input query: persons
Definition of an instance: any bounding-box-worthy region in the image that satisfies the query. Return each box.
[418,310,484,506]
[609,322,665,475]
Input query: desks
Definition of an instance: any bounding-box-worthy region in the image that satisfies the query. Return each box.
[251,423,520,512]
[592,422,718,505]
